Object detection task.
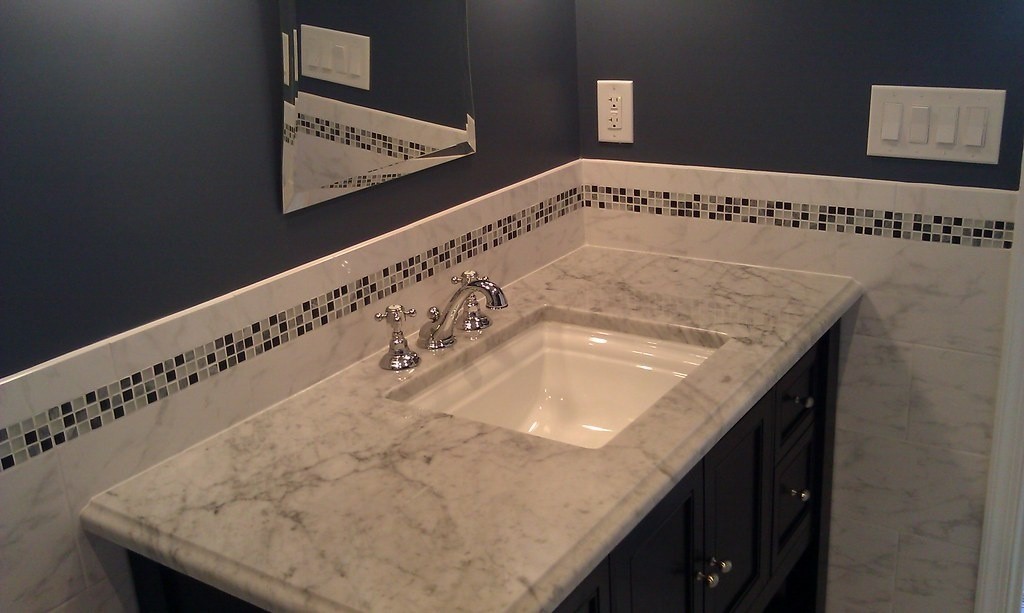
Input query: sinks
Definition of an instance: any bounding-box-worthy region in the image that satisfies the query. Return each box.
[381,305,731,453]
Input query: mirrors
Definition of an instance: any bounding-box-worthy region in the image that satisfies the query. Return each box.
[277,0,477,216]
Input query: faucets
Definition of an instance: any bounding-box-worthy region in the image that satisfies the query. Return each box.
[414,278,509,350]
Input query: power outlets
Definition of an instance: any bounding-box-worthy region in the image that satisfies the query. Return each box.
[595,80,635,145]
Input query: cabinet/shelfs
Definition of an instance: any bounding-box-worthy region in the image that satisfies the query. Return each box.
[126,317,842,613]
